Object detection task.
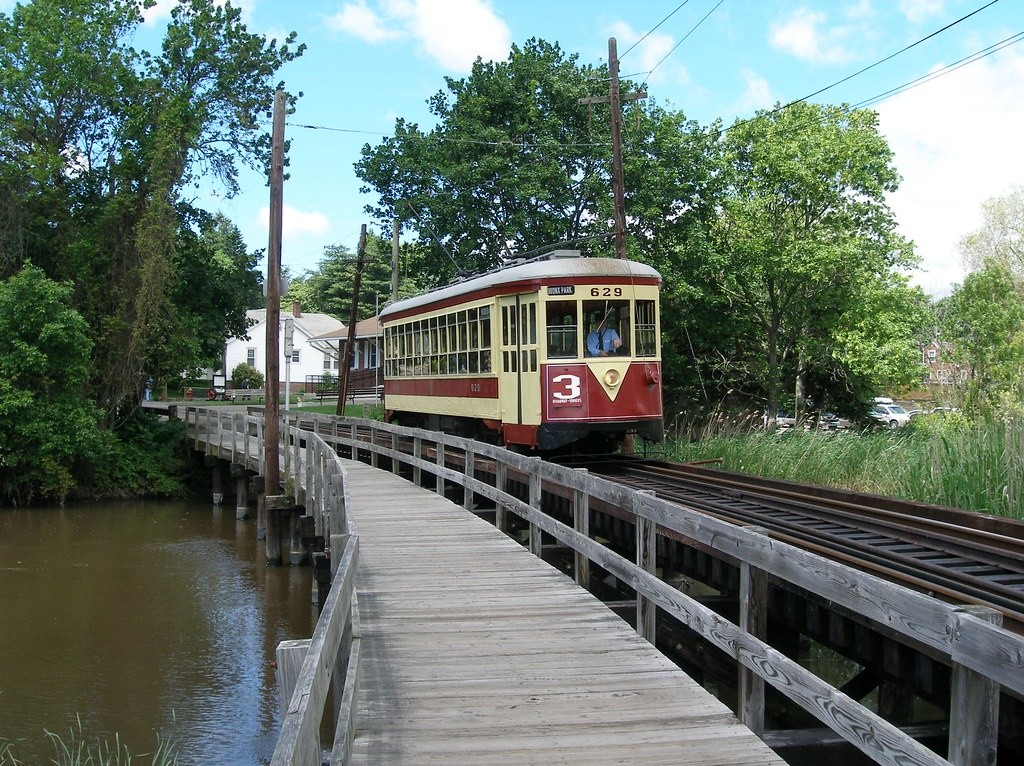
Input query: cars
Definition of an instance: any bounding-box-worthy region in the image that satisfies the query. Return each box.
[776,392,965,438]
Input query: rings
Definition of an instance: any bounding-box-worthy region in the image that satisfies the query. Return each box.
[618,342,619,344]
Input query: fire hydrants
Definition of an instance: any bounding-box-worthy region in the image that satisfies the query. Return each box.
[186,387,193,401]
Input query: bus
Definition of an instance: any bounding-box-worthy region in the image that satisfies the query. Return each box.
[377,200,667,463]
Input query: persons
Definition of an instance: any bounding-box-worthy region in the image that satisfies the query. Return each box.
[399,343,494,372]
[144,376,154,403]
[586,312,621,357]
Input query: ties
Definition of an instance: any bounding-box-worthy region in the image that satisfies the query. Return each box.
[598,331,603,351]
